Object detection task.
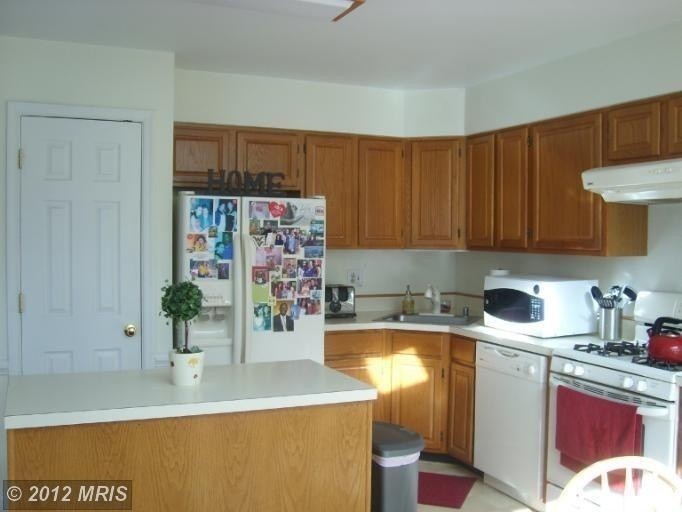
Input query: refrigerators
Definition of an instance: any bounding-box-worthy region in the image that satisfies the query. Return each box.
[175,190,325,361]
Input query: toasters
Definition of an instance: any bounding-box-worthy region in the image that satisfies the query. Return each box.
[323,284,359,320]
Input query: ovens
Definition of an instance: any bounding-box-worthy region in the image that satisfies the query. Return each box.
[546,370,681,512]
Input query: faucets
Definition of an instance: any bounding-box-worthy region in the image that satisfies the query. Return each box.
[423,283,441,313]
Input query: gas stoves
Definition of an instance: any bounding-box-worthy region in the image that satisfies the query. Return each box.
[545,338,682,402]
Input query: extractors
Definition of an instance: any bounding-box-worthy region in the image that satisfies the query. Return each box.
[581,158,682,205]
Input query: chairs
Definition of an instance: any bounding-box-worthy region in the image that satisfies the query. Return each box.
[559,456,682,512]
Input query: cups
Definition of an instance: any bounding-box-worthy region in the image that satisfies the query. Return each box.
[599,308,623,341]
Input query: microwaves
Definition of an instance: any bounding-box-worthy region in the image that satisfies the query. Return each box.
[483,276,600,339]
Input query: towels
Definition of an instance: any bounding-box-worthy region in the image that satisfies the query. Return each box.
[556,385,642,498]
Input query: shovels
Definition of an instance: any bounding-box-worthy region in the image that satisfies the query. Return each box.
[595,298,614,309]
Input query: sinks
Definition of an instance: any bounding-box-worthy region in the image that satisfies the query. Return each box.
[386,315,466,325]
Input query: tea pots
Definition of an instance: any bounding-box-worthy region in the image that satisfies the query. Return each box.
[645,316,682,366]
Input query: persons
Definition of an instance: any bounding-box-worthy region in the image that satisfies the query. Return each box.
[191,198,237,279]
[250,200,324,333]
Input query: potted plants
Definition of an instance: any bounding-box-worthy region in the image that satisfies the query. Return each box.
[158,272,208,387]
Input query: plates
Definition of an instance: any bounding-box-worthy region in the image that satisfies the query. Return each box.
[488,270,510,276]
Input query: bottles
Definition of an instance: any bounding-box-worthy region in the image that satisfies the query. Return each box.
[440,300,451,312]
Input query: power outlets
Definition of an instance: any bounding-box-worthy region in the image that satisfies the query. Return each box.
[347,270,363,287]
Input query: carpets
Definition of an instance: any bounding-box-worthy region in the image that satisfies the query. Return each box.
[417,471,478,508]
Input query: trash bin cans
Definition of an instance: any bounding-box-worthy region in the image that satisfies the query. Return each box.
[371,421,425,512]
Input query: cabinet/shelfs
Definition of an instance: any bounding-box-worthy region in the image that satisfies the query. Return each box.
[173,122,298,188]
[305,134,406,249]
[446,332,475,466]
[530,113,649,257]
[324,330,387,422]
[409,140,461,247]
[605,95,682,161]
[387,329,444,449]
[465,127,529,250]
